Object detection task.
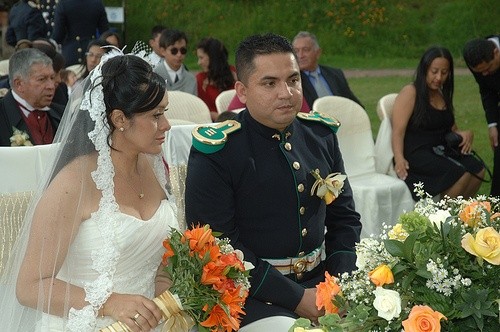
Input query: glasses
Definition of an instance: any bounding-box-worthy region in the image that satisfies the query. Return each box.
[167,47,188,55]
[85,52,102,60]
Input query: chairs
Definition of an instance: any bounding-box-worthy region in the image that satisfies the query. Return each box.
[163,89,440,244]
[0,143,61,280]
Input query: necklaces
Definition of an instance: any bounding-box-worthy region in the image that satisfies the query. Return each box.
[114,166,144,199]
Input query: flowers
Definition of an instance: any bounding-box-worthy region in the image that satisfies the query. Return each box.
[11,126,33,146]
[98,223,255,332]
[309,168,347,204]
[288,181,500,332]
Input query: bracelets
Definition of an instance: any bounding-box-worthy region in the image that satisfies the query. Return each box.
[101,305,105,320]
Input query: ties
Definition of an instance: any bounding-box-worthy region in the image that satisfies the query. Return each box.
[174,74,179,82]
[310,70,330,97]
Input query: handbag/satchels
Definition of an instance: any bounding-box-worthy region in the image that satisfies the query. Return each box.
[431,131,494,183]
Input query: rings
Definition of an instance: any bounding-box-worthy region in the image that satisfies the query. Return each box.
[133,313,140,320]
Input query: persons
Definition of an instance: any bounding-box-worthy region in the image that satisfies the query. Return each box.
[0,0,364,332]
[390,34,500,203]
